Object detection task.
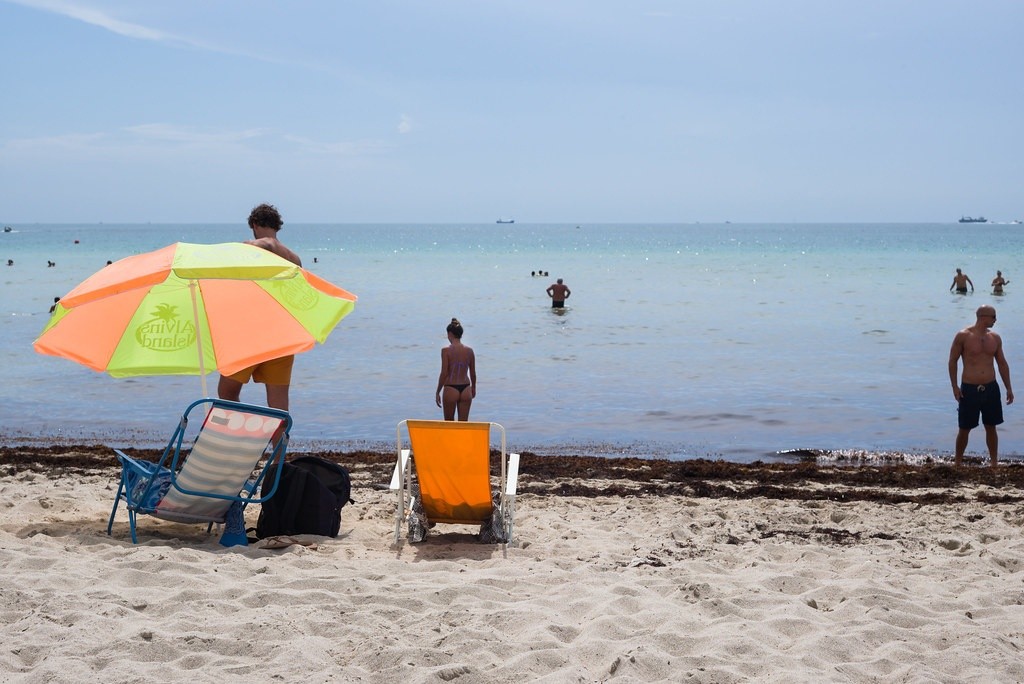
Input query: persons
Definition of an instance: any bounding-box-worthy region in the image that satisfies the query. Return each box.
[992,270,1010,294]
[212,203,304,468]
[546,278,571,308]
[950,267,974,292]
[947,304,1014,468]
[435,315,479,422]
[48,297,60,314]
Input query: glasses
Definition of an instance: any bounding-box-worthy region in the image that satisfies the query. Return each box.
[980,315,996,319]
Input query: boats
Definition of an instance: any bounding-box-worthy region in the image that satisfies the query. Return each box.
[496,219,515,223]
[959,214,987,223]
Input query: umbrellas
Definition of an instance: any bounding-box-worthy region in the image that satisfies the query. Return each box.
[34,240,360,442]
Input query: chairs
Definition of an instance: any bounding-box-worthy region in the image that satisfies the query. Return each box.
[389,419,519,546]
[108,397,292,548]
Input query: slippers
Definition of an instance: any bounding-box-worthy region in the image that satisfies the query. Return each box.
[258,536,317,550]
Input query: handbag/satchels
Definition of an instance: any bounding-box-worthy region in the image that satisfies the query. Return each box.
[244,457,355,544]
